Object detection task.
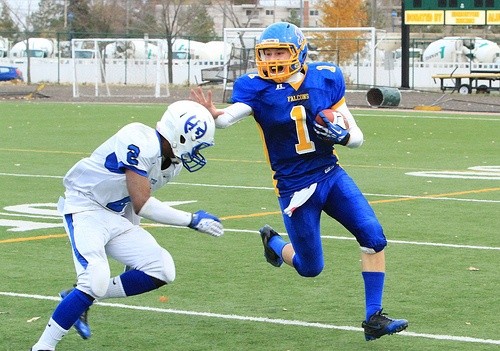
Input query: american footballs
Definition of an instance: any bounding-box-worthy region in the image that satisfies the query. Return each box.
[314,109,349,144]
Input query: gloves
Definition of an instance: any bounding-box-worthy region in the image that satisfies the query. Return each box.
[314,112,350,148]
[188,210,225,237]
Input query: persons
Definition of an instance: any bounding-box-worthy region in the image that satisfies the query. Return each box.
[191,21,409,342]
[28,99,223,351]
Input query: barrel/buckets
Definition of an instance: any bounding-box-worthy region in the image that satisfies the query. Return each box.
[367,88,401,109]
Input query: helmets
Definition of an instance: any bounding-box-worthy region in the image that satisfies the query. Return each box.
[257,21,309,84]
[155,98,215,163]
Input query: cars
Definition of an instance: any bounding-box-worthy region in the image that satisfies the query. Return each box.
[0,65,24,82]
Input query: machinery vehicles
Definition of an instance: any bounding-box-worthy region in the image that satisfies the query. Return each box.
[0,37,237,62]
[393,36,500,68]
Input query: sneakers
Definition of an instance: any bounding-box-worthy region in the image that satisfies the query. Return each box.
[59,284,91,340]
[259,225,284,269]
[361,308,408,341]
